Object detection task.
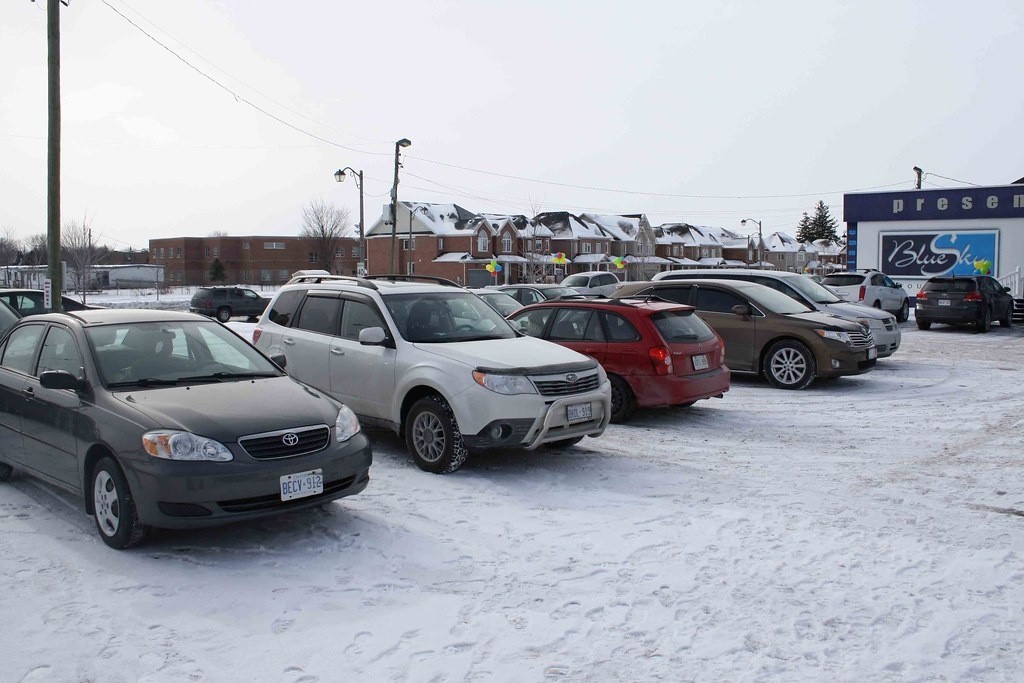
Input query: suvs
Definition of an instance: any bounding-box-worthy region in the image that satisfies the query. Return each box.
[247,265,613,477]
[649,269,903,361]
[189,286,277,324]
[817,268,911,323]
[484,292,731,424]
[605,278,878,394]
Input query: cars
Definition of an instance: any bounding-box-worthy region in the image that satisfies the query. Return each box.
[479,283,592,330]
[0,298,57,358]
[915,275,1015,334]
[394,286,547,334]
[0,308,374,551]
[0,286,113,320]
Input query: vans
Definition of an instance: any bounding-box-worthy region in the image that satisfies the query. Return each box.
[559,270,623,302]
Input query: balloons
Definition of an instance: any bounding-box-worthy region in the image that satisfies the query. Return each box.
[803,266,812,273]
[552,252,566,263]
[973,261,992,275]
[486,260,501,276]
[614,257,628,269]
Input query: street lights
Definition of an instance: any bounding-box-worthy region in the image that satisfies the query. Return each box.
[739,218,763,271]
[389,138,412,280]
[334,166,368,275]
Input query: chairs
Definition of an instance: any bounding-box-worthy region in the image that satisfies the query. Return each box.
[57,326,169,387]
[557,322,578,339]
[407,302,436,339]
